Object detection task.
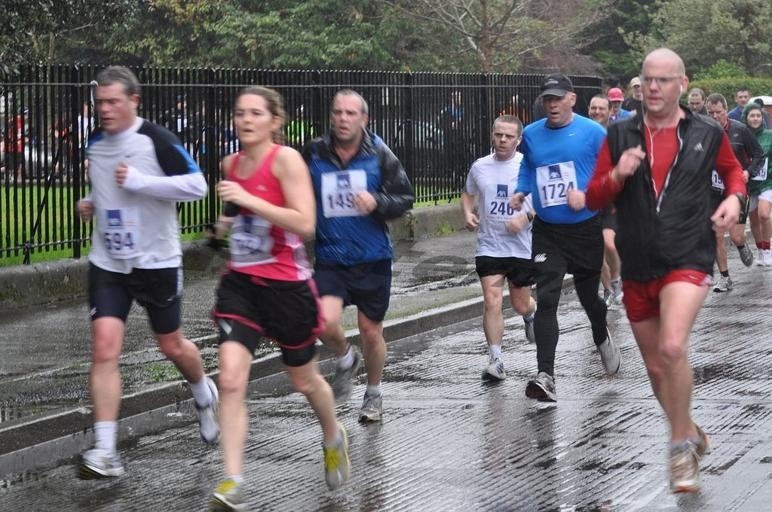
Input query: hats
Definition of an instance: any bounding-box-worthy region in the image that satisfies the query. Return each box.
[607,88,625,102]
[630,78,641,88]
[538,73,576,101]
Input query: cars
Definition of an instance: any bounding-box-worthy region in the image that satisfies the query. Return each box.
[0,134,65,179]
[362,119,465,175]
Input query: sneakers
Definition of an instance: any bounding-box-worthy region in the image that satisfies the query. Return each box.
[756,247,772,267]
[526,371,557,403]
[208,480,248,509]
[738,242,754,267]
[319,424,351,493]
[600,283,624,310]
[359,393,385,426]
[334,348,363,397]
[712,276,733,293]
[74,449,124,479]
[598,327,620,376]
[523,319,537,342]
[195,380,221,446]
[483,357,507,382]
[668,421,709,495]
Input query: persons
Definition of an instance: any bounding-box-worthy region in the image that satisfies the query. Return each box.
[437,89,464,131]
[509,73,620,402]
[459,114,536,380]
[76,66,221,480]
[4,94,314,183]
[586,77,644,310]
[586,47,747,493]
[686,87,771,291]
[209,84,350,510]
[299,90,415,423]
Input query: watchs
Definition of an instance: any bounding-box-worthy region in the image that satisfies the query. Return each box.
[527,212,533,222]
